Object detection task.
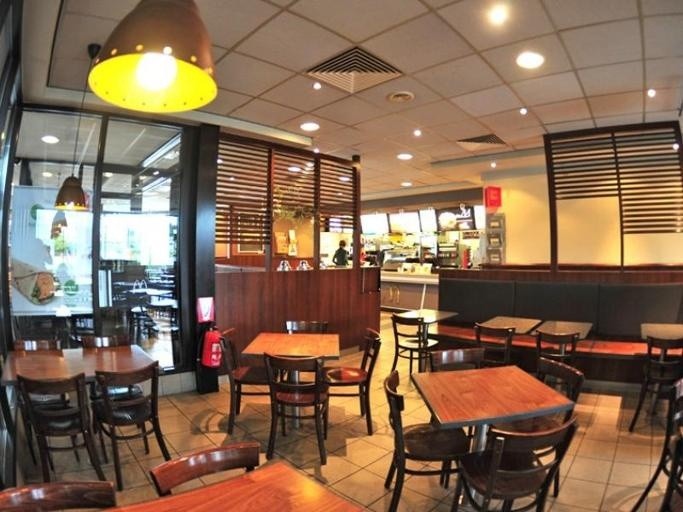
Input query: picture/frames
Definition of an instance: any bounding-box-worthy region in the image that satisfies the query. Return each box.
[237,213,265,253]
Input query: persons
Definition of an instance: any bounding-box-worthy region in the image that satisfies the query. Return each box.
[331,239,350,269]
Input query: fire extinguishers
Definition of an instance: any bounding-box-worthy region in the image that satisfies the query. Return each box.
[196,321,223,368]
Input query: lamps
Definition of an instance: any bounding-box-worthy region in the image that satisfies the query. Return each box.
[55,44,102,212]
[86,0,218,113]
